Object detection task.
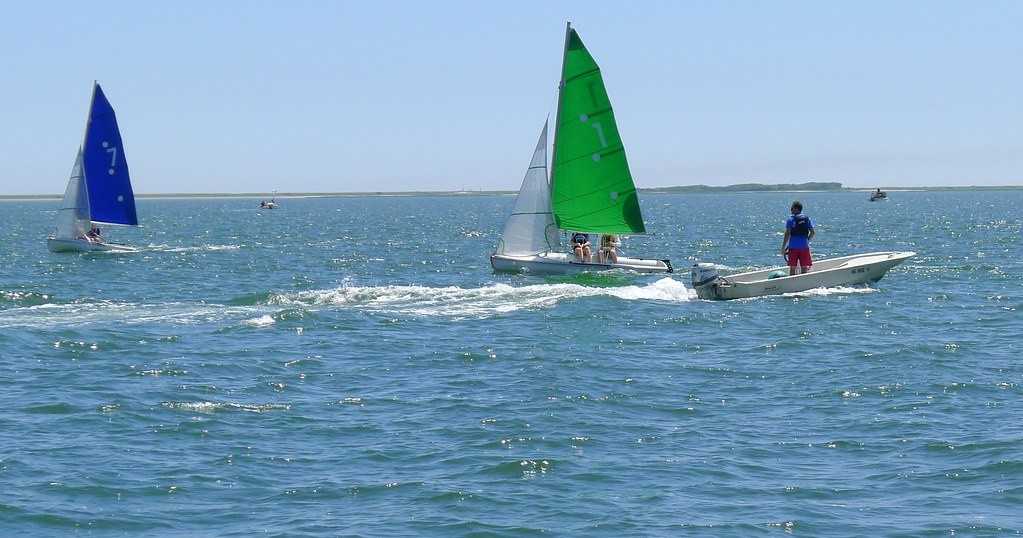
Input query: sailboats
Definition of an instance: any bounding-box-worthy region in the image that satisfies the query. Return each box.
[47,80,138,253]
[490,21,674,272]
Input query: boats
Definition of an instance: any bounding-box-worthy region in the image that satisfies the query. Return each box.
[260,203,278,209]
[692,250,918,301]
[868,192,888,202]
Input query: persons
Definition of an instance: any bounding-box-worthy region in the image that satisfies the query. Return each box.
[596,233,621,264]
[570,232,593,263]
[261,201,265,207]
[781,202,815,275]
[86,223,100,239]
